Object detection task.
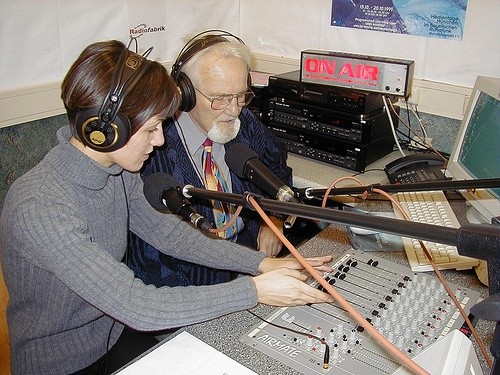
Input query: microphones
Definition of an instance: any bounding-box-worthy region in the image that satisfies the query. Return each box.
[224,142,300,203]
[143,172,212,232]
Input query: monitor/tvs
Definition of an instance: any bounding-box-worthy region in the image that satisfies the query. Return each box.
[445,76,500,224]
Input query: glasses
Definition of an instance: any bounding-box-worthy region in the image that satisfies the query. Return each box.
[193,88,255,110]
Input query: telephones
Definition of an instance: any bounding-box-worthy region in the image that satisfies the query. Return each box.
[384,153,451,184]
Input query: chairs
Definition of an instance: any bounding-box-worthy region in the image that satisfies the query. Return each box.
[0,112,71,216]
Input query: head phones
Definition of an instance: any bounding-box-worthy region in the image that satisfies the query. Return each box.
[171,30,253,112]
[76,37,155,153]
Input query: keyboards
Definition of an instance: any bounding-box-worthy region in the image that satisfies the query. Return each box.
[390,190,478,273]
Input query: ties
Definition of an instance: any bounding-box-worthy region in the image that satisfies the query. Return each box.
[201,138,238,244]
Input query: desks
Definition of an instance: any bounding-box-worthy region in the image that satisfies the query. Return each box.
[117,150,500,375]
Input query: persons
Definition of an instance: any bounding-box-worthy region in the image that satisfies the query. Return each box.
[127,29,308,291]
[0,40,337,375]
